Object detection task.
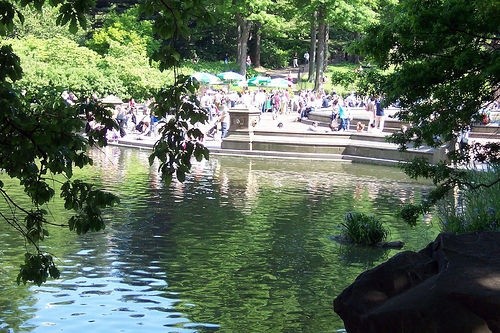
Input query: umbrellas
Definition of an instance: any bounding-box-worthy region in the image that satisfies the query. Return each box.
[192,72,291,89]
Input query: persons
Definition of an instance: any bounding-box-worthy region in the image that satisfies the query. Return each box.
[446,130,458,165]
[62,88,368,144]
[376,97,387,131]
[367,96,376,128]
[247,55,253,66]
[304,51,310,64]
[293,52,298,68]
[458,129,470,161]
[398,123,408,134]
[224,53,230,61]
